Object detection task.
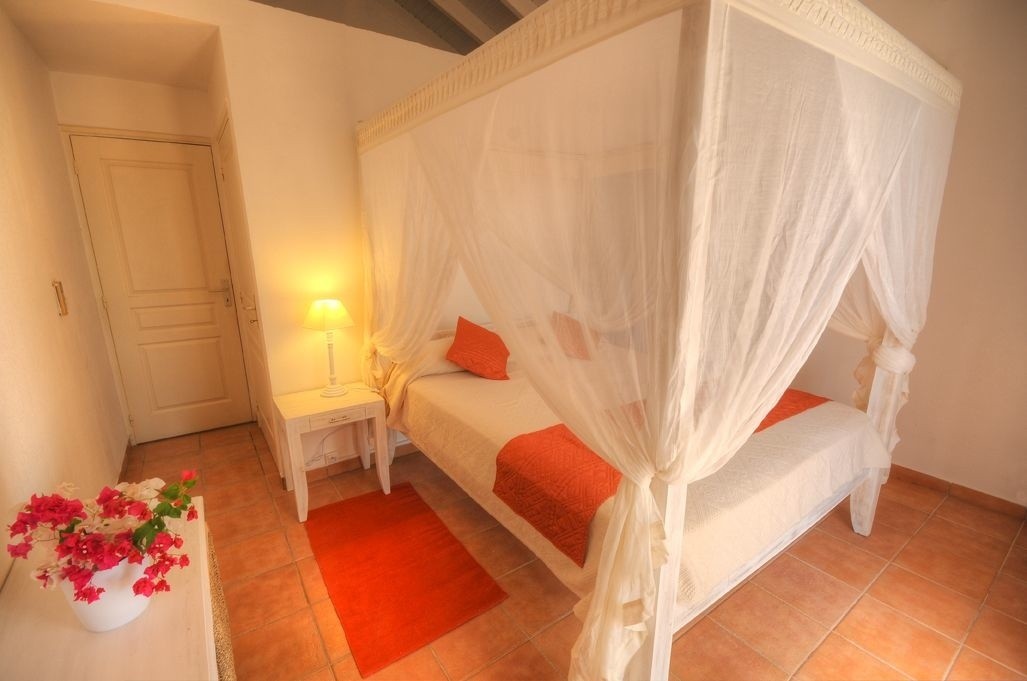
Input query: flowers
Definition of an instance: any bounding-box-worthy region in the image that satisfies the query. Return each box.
[5,465,200,606]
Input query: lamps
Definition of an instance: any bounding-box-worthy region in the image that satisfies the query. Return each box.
[303,299,353,398]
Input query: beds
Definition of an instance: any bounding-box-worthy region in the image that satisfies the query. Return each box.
[354,1,966,681]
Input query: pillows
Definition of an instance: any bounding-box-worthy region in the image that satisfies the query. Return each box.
[546,310,600,357]
[446,316,513,380]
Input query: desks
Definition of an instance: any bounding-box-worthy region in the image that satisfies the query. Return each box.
[0,496,237,681]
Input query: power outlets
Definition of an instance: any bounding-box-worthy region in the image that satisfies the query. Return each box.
[324,450,339,464]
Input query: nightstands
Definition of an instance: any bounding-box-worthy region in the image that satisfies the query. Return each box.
[271,377,392,524]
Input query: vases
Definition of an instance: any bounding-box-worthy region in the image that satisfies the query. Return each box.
[60,550,151,634]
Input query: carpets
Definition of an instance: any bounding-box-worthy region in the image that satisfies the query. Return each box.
[304,479,511,679]
